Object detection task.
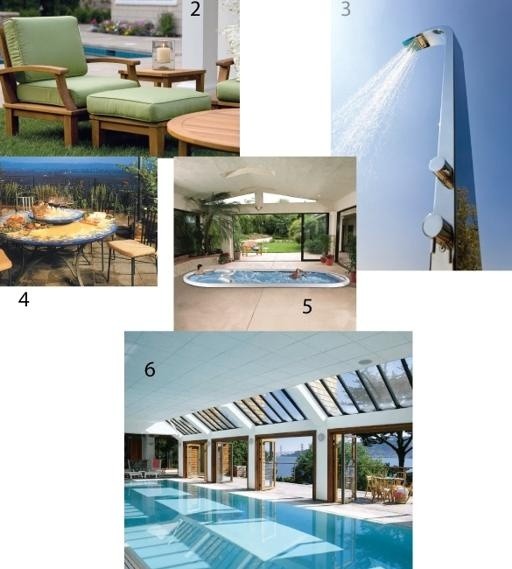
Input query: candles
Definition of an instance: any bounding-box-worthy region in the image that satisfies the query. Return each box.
[157,44,170,63]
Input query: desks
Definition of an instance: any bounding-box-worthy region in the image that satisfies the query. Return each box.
[118,67,207,93]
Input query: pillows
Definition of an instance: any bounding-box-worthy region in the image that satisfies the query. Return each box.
[2,16,88,82]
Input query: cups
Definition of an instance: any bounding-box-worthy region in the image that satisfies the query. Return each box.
[151,39,176,71]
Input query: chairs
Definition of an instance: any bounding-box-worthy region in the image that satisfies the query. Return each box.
[365,472,412,504]
[107,208,158,286]
[125,459,162,478]
[0,16,141,147]
[0,249,13,286]
[16,195,34,211]
[211,55,240,109]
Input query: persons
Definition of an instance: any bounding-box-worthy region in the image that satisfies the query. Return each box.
[290,268,303,280]
[194,263,206,275]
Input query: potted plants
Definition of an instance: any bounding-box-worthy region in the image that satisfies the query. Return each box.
[344,233,356,282]
[319,234,334,266]
[235,234,249,259]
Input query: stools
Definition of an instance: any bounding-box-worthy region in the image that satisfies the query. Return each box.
[87,87,211,157]
